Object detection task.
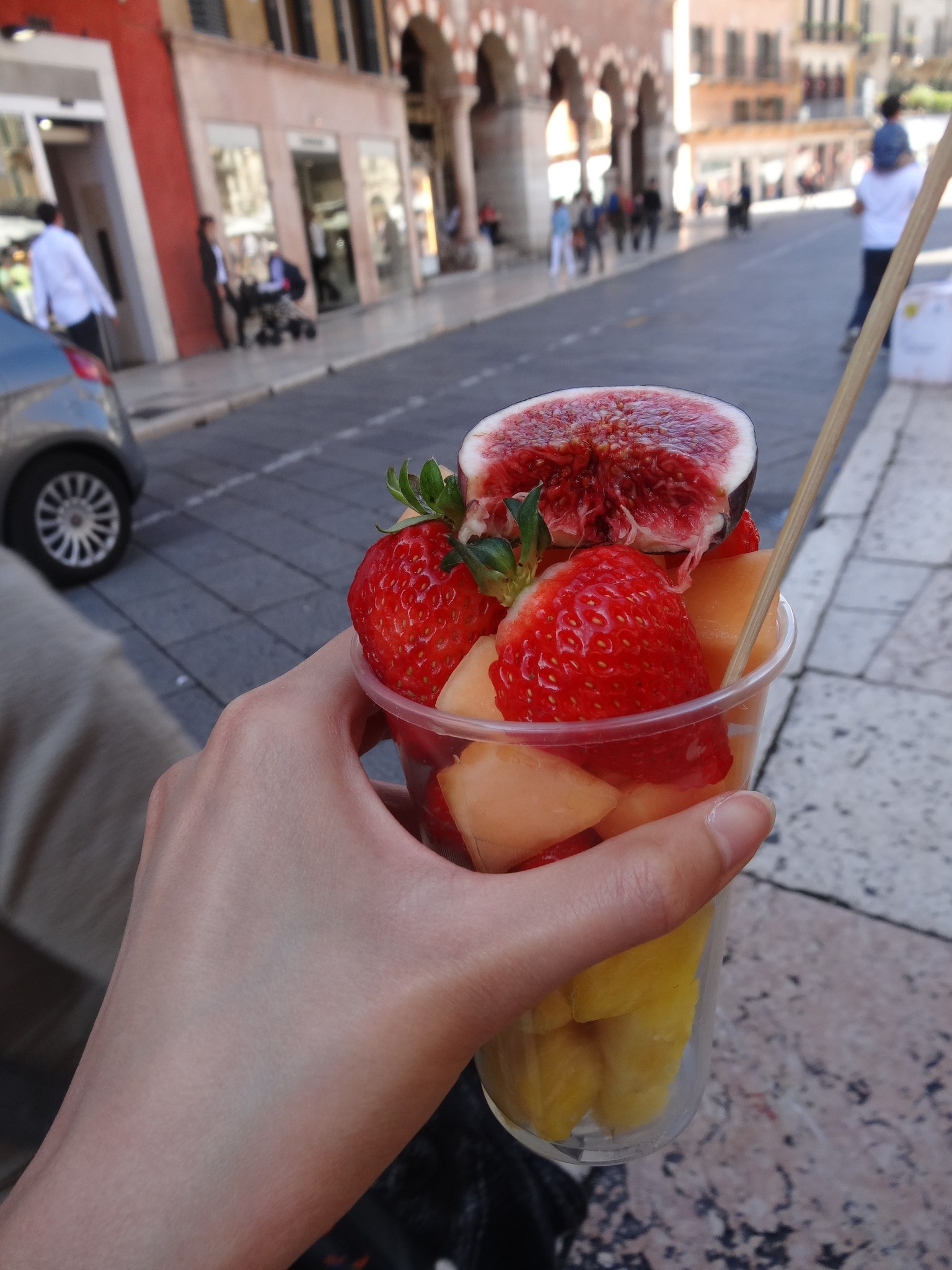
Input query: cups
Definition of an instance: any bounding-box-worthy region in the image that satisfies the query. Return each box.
[350,594,798,1166]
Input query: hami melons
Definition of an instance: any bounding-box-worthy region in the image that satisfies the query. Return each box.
[429,550,780,875]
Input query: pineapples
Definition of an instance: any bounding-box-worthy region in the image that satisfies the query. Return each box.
[471,899,717,1142]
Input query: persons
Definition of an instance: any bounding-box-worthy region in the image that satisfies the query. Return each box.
[197,217,245,349]
[548,192,603,275]
[609,176,661,253]
[480,202,501,245]
[302,207,339,303]
[843,95,923,353]
[0,541,774,1269]
[29,200,120,353]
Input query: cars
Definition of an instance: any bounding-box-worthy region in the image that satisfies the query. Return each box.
[0,310,146,587]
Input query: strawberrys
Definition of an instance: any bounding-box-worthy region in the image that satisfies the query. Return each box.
[441,477,734,785]
[665,508,761,569]
[347,459,507,768]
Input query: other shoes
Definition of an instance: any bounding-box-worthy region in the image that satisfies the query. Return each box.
[843,328,859,354]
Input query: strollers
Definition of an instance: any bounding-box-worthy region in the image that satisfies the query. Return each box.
[226,252,321,346]
